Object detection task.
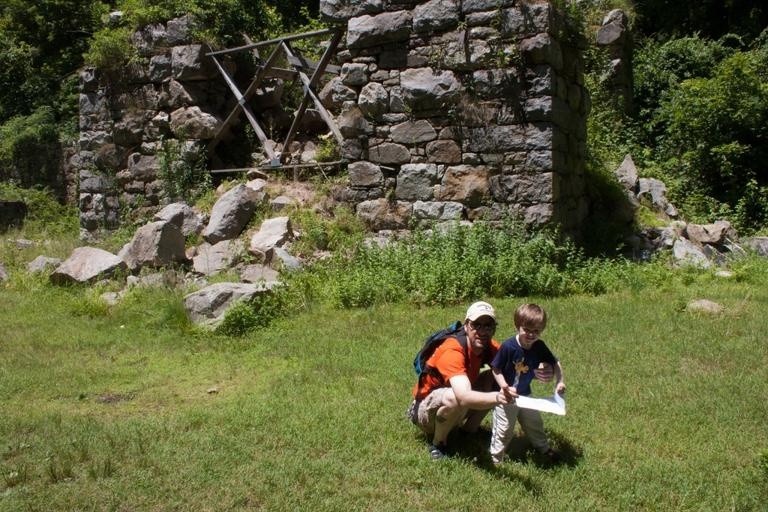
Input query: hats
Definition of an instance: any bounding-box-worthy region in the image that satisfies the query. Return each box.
[465,300,496,325]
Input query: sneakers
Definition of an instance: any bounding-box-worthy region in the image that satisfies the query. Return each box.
[491,454,503,464]
[429,445,443,462]
[455,427,483,442]
[538,447,558,461]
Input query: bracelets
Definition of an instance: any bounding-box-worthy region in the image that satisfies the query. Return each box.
[494,392,501,404]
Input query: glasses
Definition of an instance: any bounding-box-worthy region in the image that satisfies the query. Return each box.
[470,321,493,331]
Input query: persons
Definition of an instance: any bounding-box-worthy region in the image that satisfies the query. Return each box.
[487,302,567,469]
[407,300,555,462]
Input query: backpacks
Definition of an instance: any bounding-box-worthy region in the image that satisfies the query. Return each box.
[413,320,489,383]
